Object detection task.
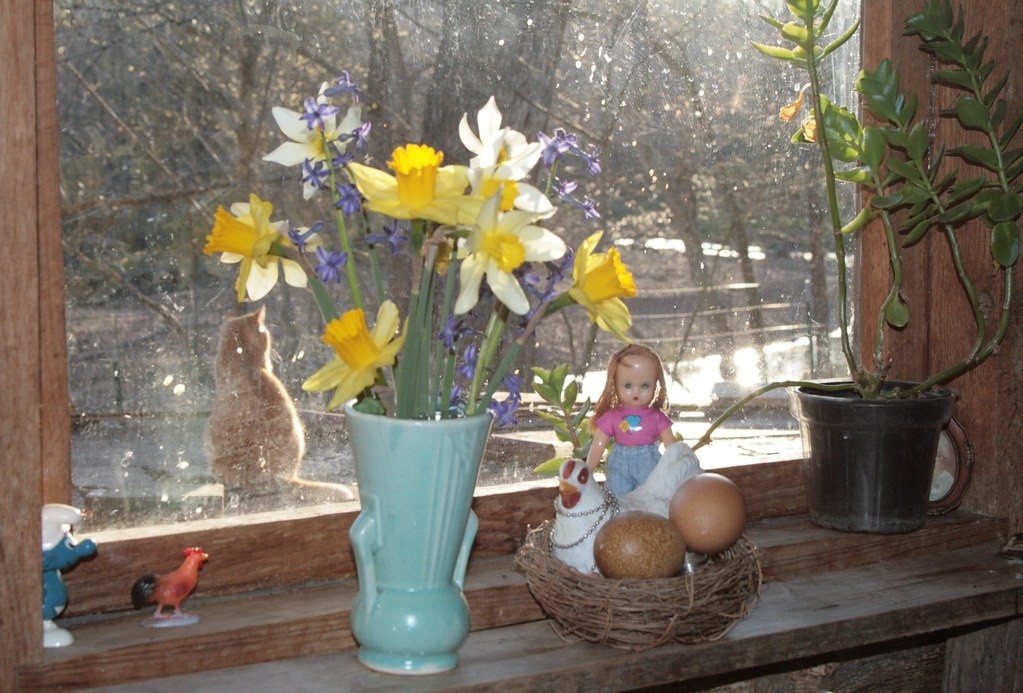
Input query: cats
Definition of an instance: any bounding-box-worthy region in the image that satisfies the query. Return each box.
[203,305,353,504]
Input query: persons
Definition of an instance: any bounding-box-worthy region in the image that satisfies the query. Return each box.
[42,504,96,647]
[584,343,676,499]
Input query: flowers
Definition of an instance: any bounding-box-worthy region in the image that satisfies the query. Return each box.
[203,68,637,410]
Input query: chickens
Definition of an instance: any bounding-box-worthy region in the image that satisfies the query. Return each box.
[551,440,703,575]
[129,546,211,619]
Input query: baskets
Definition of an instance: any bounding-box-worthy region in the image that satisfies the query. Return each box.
[516,519,762,651]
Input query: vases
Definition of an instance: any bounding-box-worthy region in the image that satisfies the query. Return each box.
[344,396,496,676]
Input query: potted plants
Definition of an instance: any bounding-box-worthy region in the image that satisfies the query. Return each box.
[688,0,1023,535]
